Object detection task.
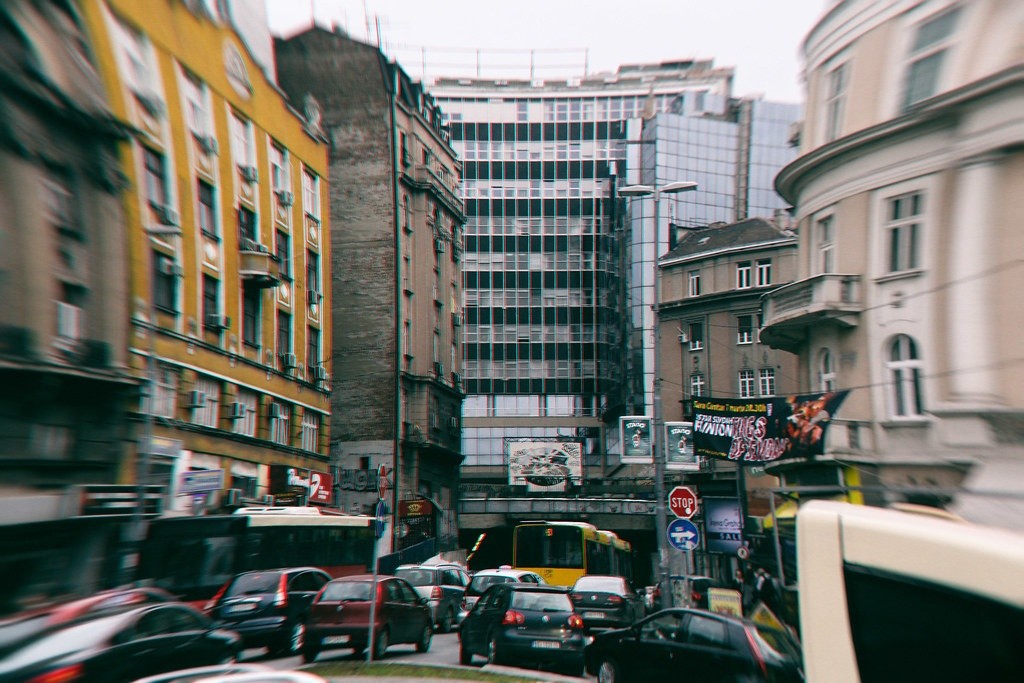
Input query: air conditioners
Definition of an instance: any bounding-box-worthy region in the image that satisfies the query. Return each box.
[283,353,296,366]
[158,204,179,225]
[228,402,245,416]
[203,135,218,151]
[209,314,231,328]
[455,373,461,381]
[435,240,444,251]
[281,191,293,203]
[268,403,280,415]
[245,166,258,180]
[454,315,461,325]
[436,170,443,179]
[242,238,255,250]
[314,366,325,379]
[186,390,206,405]
[435,362,443,373]
[255,244,268,253]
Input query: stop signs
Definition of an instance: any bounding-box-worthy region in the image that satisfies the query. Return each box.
[667,484,699,519]
[376,464,388,502]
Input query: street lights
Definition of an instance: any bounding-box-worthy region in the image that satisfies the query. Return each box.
[612,178,700,624]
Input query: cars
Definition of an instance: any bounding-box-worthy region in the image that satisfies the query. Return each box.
[396,561,472,633]
[0,584,180,646]
[204,565,334,656]
[0,598,244,682]
[298,571,434,664]
[457,565,547,626]
[454,579,591,676]
[581,607,804,683]
[564,574,652,630]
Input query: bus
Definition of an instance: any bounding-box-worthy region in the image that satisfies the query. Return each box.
[511,520,636,586]
[138,507,393,603]
